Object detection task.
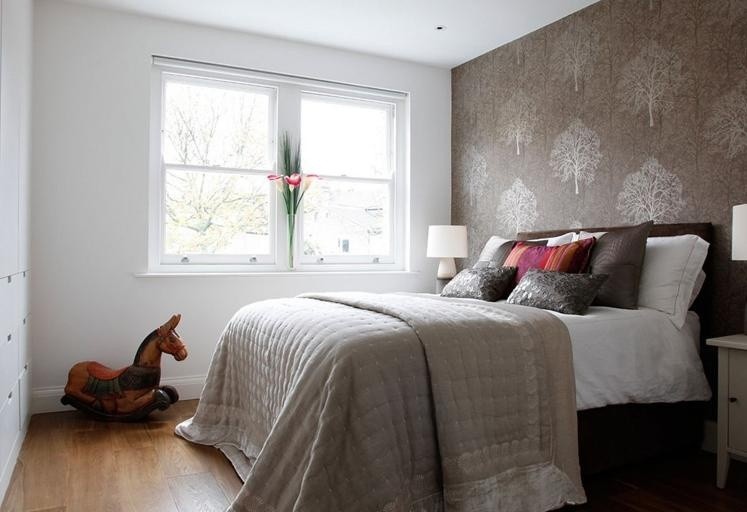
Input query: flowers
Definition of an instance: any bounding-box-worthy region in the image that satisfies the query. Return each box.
[266,131,322,267]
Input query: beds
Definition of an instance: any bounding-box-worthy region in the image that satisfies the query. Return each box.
[172,220,714,511]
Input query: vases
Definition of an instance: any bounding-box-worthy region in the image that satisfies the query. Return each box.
[286,215,296,268]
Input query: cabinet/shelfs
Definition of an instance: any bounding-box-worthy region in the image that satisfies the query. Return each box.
[0,1,33,511]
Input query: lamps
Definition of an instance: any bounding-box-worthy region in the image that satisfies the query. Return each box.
[731,205,745,260]
[427,225,467,279]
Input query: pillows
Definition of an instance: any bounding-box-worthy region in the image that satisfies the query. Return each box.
[491,239,548,264]
[583,220,656,309]
[638,234,711,329]
[441,266,517,301]
[477,232,576,262]
[502,237,597,281]
[506,266,608,315]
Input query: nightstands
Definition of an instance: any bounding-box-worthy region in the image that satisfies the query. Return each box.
[706,336,747,489]
